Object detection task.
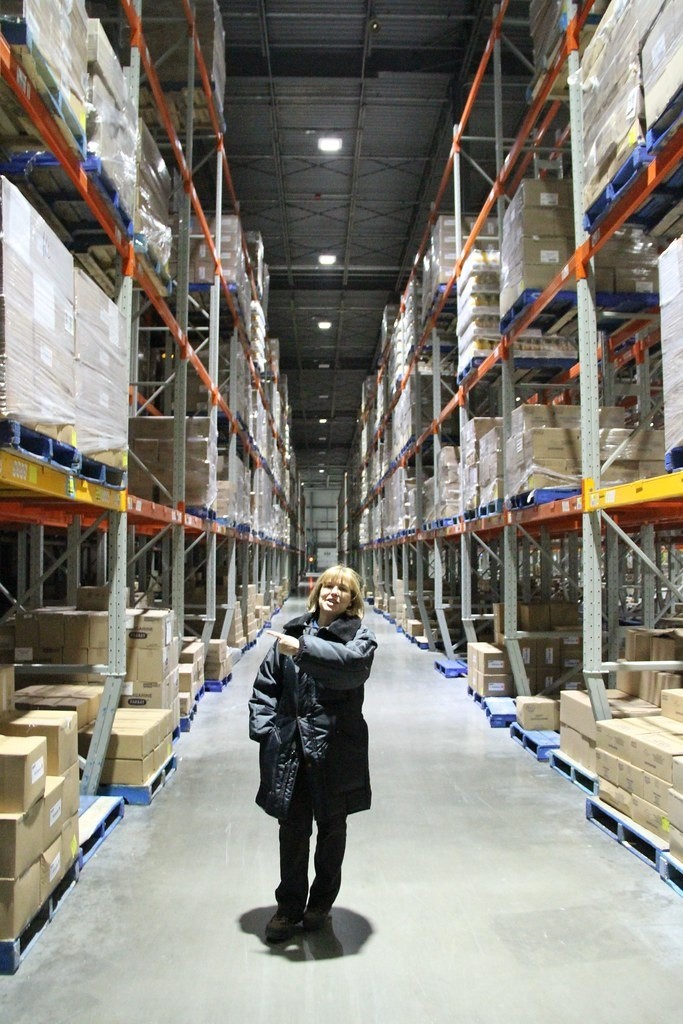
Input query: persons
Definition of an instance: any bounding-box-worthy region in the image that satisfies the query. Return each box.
[248,565,378,940]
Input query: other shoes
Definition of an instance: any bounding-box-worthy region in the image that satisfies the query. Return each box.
[266,910,302,946]
[304,906,331,925]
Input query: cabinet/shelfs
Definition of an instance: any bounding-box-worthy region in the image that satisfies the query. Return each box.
[359,0,683,896]
[0,1,302,976]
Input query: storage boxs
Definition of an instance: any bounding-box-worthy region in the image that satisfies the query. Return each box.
[0,35,293,936]
[361,0,683,863]
